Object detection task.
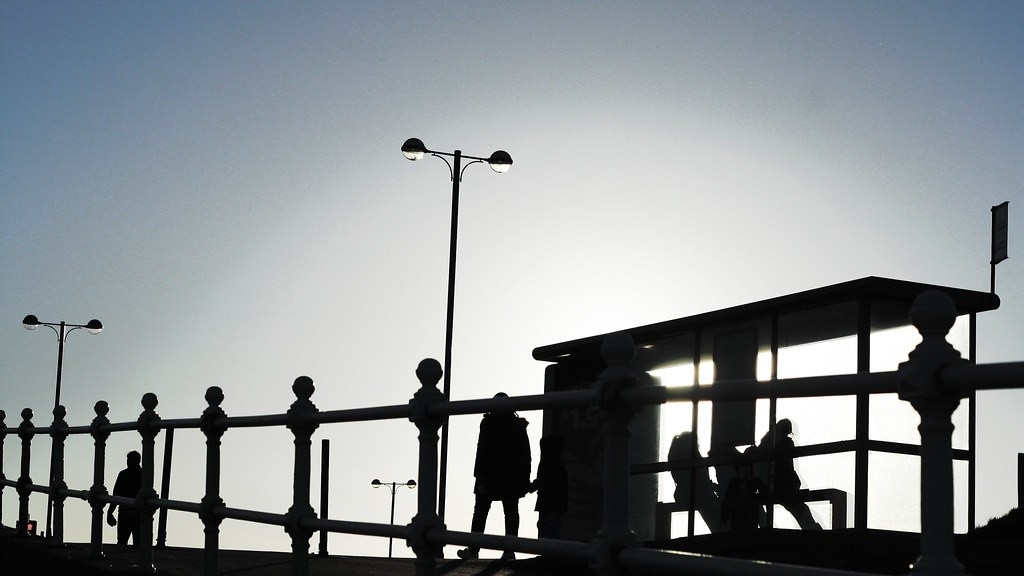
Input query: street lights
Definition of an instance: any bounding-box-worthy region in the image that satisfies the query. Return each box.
[369,478,417,559]
[22,313,106,545]
[399,137,516,557]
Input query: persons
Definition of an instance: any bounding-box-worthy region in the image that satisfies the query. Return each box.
[668,431,718,534]
[457,392,531,560]
[107,450,159,545]
[760,419,822,530]
[529,432,568,540]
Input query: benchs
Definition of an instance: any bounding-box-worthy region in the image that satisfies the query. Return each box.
[654,488,847,542]
